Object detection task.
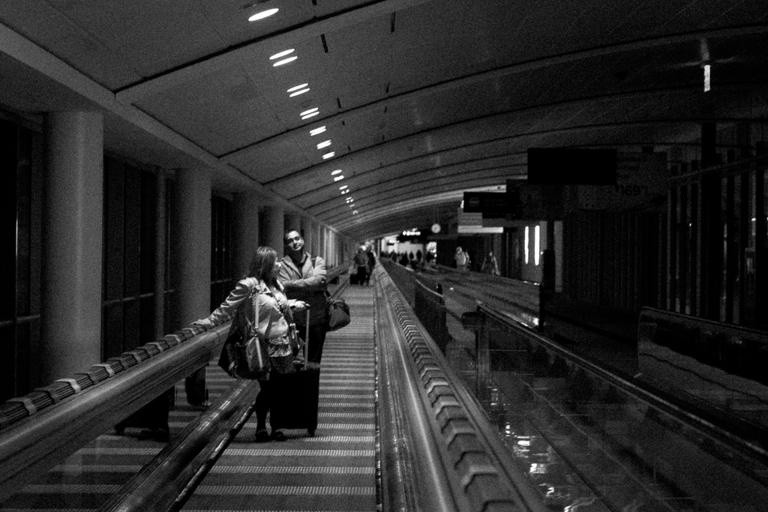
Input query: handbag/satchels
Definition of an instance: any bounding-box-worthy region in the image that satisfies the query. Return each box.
[326,297,351,331]
[222,338,265,377]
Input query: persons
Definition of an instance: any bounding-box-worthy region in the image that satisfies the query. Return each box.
[381,248,435,272]
[480,250,498,276]
[354,245,375,287]
[455,246,469,272]
[188,246,306,441]
[277,228,327,364]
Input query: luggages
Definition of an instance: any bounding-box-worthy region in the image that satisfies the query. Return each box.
[269,304,320,431]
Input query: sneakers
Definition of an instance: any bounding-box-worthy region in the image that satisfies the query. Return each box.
[254,429,285,443]
